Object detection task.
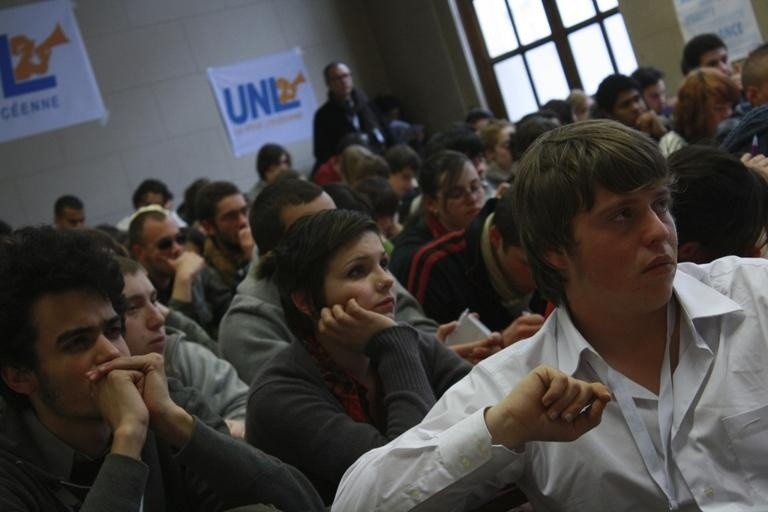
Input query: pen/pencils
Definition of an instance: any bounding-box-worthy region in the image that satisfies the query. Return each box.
[577,402,593,417]
[751,134,759,158]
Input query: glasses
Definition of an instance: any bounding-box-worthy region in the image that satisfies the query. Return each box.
[147,232,189,248]
